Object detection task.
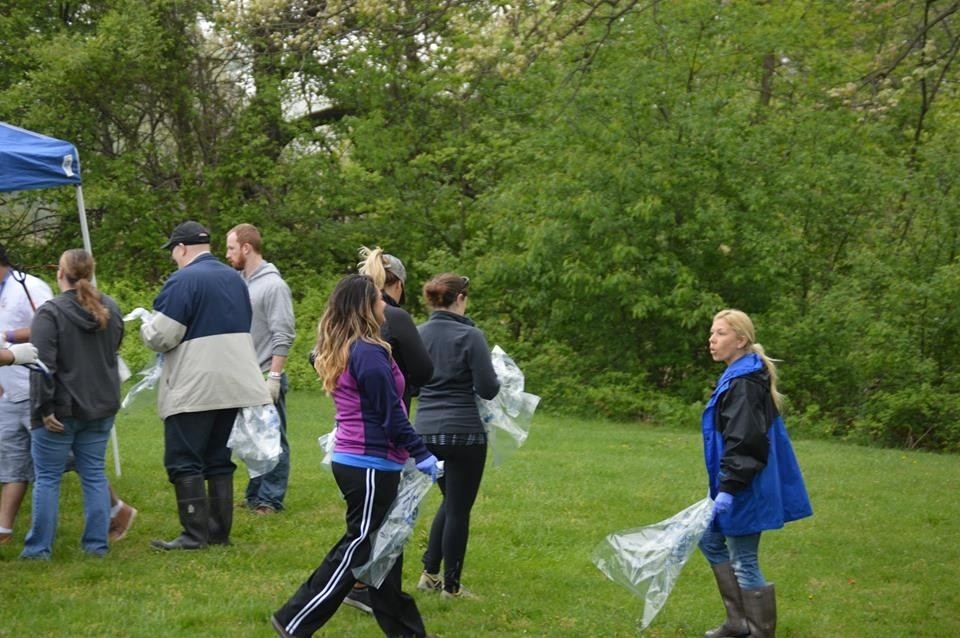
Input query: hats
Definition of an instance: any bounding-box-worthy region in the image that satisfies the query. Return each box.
[384,254,406,306]
[161,221,211,251]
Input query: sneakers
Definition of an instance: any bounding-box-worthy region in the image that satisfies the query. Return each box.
[440,583,478,600]
[415,569,443,591]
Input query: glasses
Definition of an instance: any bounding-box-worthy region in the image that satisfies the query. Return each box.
[461,276,470,289]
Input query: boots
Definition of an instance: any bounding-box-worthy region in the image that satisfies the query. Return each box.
[704,561,751,638]
[744,582,777,638]
[149,474,211,550]
[207,473,235,544]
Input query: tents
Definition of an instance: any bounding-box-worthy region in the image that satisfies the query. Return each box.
[0,119,124,478]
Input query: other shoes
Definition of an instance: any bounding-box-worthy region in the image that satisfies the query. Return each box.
[256,506,275,515]
[232,499,249,509]
[108,503,137,542]
[343,585,372,613]
[269,615,298,638]
[0,531,15,544]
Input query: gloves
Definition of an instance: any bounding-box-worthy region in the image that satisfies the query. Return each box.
[266,370,280,405]
[714,491,735,514]
[416,455,439,485]
[8,342,39,367]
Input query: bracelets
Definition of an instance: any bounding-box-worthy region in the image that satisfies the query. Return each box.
[269,370,281,377]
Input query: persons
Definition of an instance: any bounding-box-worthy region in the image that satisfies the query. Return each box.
[341,244,435,612]
[0,241,138,543]
[413,274,501,603]
[268,275,432,638]
[140,221,273,553]
[224,222,297,517]
[17,249,126,562]
[698,310,813,638]
